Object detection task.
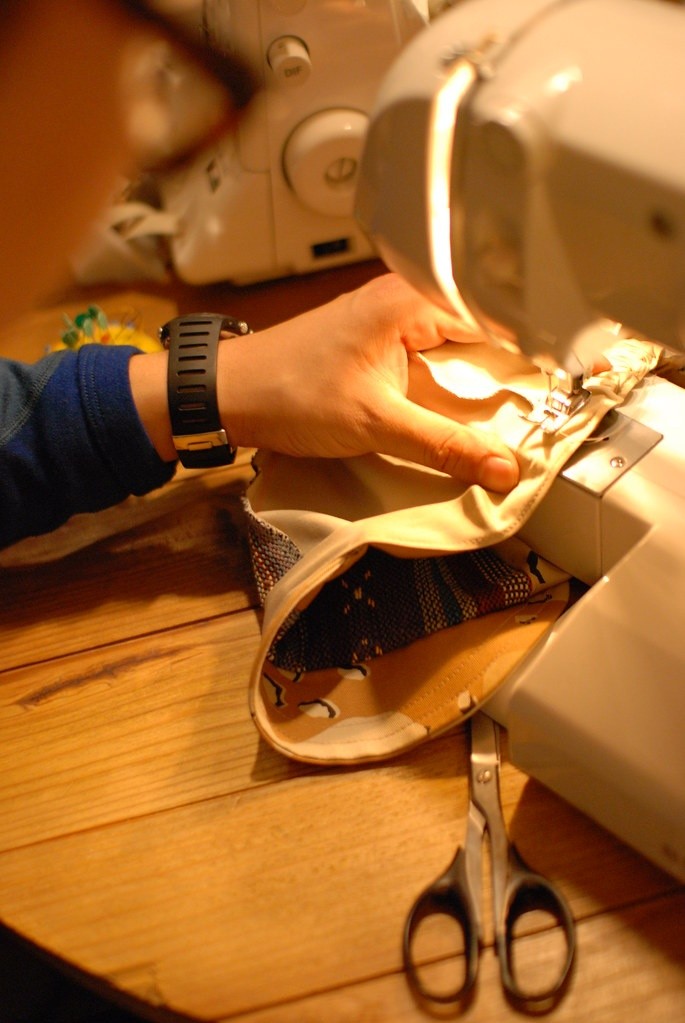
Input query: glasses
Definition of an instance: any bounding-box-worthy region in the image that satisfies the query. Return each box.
[127,2,259,180]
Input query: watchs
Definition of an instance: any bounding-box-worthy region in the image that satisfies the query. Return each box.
[156,313,254,469]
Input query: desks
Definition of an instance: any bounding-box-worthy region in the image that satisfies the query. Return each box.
[0,258,685,1022]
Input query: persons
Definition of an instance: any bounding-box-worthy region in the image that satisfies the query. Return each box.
[0,273,520,550]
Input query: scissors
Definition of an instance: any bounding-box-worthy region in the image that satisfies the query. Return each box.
[400,707,578,1004]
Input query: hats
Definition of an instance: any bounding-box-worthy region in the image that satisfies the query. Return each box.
[244,330,685,769]
